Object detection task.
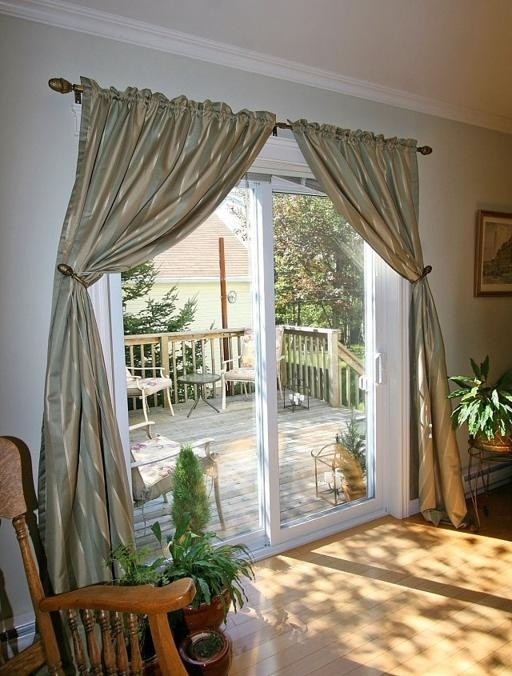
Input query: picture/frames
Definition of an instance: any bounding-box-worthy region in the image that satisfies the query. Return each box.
[470,206,511,298]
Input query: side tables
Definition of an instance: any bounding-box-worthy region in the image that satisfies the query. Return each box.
[176,373,224,418]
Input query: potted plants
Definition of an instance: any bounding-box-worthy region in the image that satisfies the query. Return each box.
[103,443,256,676]
[449,353,512,446]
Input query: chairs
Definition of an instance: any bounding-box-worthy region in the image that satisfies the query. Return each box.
[124,366,175,439]
[220,324,287,410]
[122,422,227,534]
[0,435,204,676]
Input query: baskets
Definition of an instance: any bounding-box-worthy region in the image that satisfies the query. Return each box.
[469,420,512,452]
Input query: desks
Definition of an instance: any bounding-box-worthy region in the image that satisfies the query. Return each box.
[465,439,512,535]
